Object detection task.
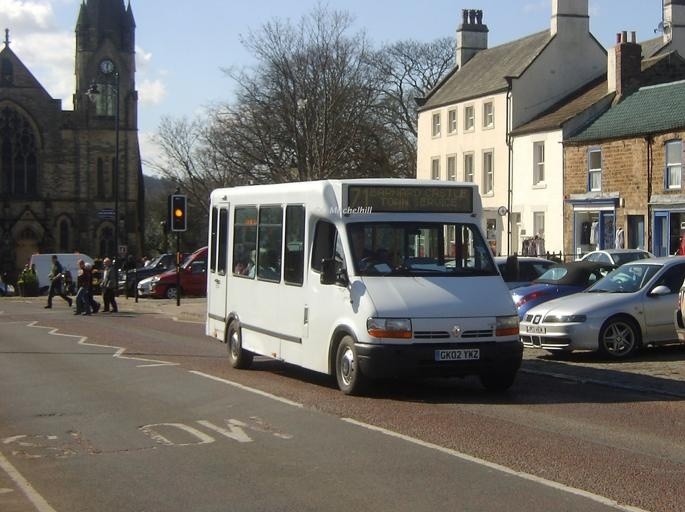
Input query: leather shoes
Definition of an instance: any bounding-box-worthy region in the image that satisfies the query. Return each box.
[73,303,119,315]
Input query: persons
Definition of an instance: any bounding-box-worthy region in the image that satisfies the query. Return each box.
[18,263,38,283]
[45,255,72,308]
[236,237,408,277]
[64,254,148,315]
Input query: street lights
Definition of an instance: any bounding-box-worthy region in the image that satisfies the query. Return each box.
[86,73,120,297]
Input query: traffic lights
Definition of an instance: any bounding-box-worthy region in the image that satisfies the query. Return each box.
[171,195,187,231]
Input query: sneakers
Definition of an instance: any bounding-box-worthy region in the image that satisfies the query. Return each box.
[45,305,51,308]
[69,299,72,306]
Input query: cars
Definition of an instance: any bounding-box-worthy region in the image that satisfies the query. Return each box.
[117,246,208,299]
[445,249,685,361]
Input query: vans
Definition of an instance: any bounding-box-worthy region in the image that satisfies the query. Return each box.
[30,254,93,295]
[205,178,524,396]
